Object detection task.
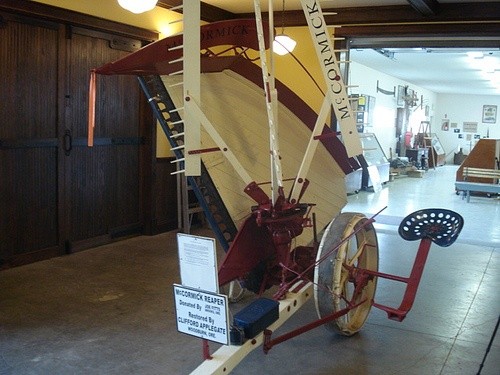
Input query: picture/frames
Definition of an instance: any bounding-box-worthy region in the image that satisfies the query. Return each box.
[482,105,497,123]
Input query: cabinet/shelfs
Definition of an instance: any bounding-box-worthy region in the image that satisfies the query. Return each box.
[417,132,446,166]
[455,139,500,198]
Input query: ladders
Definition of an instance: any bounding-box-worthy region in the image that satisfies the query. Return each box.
[136,73,239,254]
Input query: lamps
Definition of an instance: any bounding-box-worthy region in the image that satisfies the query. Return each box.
[271,1,296,55]
